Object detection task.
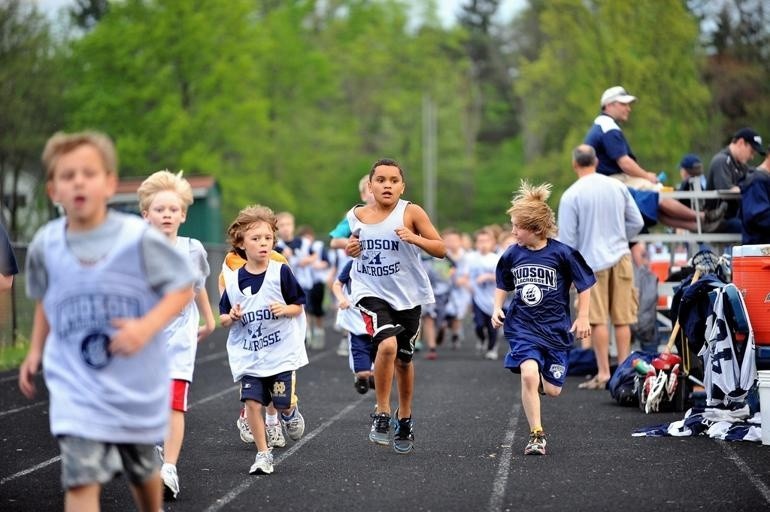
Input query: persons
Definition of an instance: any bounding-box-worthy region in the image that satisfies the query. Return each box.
[448,225,490,360]
[702,127,766,234]
[558,144,644,390]
[490,179,598,456]
[490,222,518,360]
[0,221,19,290]
[218,203,309,476]
[586,85,728,232]
[217,246,291,449]
[670,154,707,235]
[414,225,448,360]
[327,247,352,357]
[332,257,376,394]
[18,130,196,511]
[294,223,331,351]
[735,143,770,245]
[346,158,448,454]
[136,168,216,502]
[328,173,378,251]
[275,212,317,351]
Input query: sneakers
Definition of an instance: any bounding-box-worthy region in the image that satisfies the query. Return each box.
[237,408,254,442]
[160,464,180,496]
[702,219,721,232]
[355,372,369,394]
[705,201,728,219]
[525,429,547,454]
[392,407,414,453]
[266,421,285,446]
[369,374,376,389]
[249,452,273,474]
[277,407,304,440]
[369,404,392,445]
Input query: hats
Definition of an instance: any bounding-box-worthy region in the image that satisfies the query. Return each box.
[601,86,638,106]
[734,127,766,157]
[680,154,702,169]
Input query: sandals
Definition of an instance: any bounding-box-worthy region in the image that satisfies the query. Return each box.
[578,375,606,389]
